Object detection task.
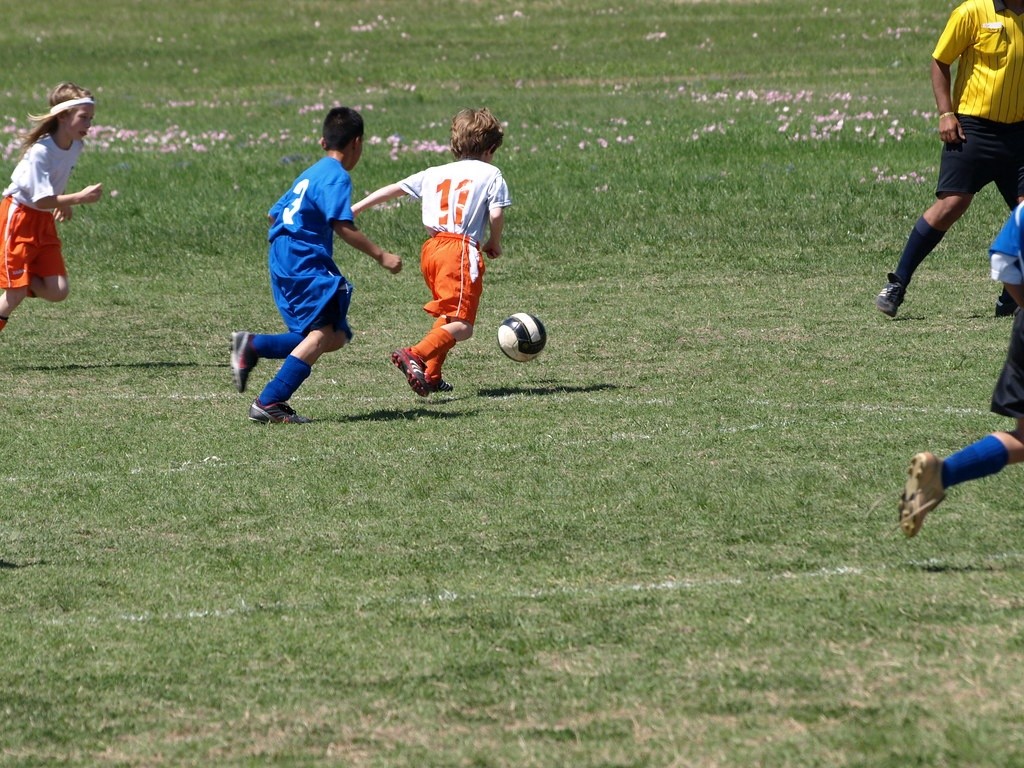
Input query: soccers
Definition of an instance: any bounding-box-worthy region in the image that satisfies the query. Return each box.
[497,312,547,362]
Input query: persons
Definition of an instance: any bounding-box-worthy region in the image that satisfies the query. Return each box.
[0,83,103,333]
[899,201,1024,538]
[350,108,511,398]
[229,107,403,425]
[877,1,1024,318]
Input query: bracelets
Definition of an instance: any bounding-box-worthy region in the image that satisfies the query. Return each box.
[939,112,954,119]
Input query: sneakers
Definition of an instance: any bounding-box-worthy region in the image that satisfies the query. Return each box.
[247,397,311,425]
[895,452,945,539]
[391,346,430,398]
[226,330,259,393]
[875,272,907,316]
[424,373,453,392]
[994,284,1021,316]
